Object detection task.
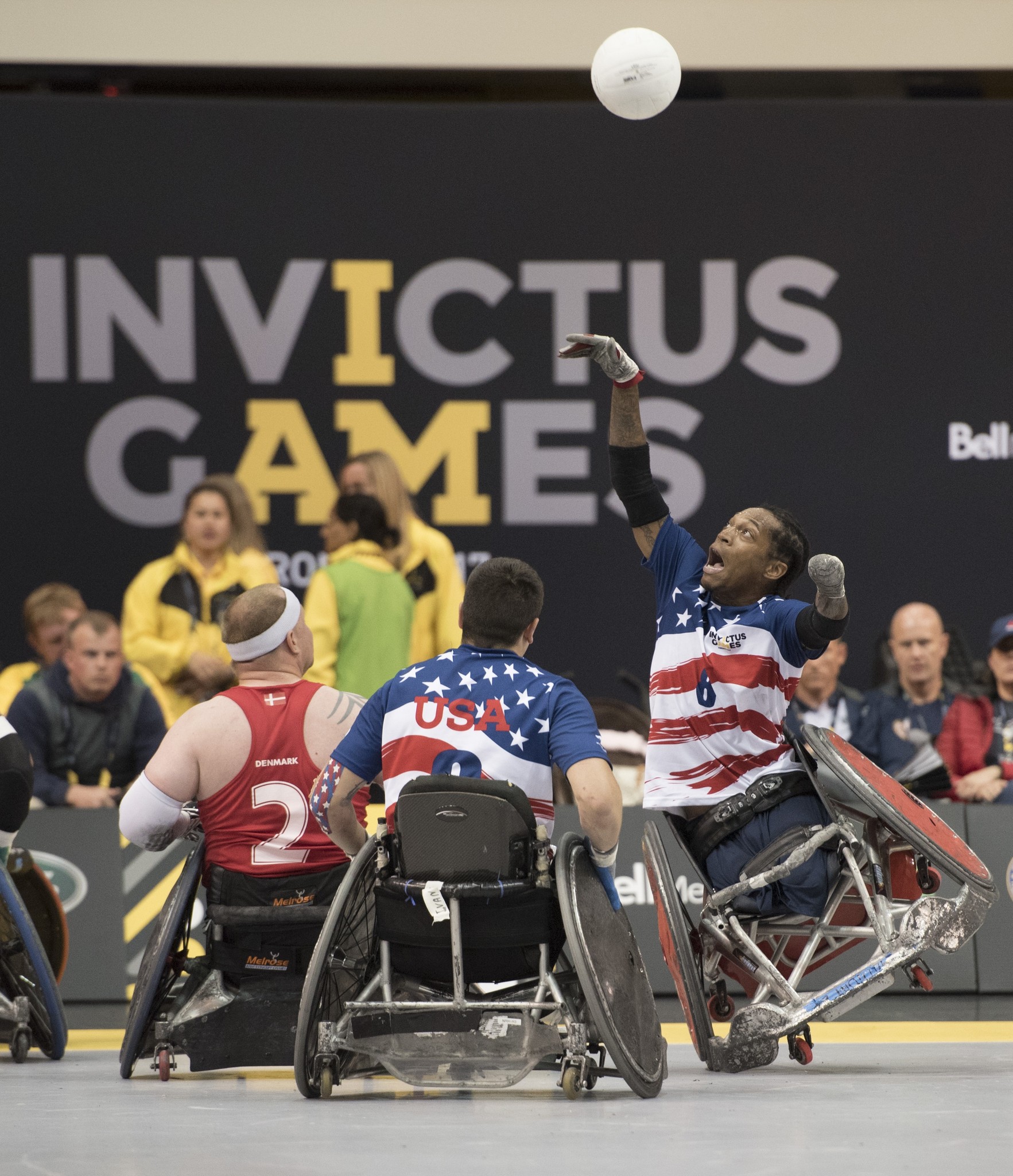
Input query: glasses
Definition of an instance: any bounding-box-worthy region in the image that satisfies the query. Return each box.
[996,634,1013,652]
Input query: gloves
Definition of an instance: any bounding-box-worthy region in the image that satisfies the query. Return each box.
[589,839,621,911]
[557,333,646,390]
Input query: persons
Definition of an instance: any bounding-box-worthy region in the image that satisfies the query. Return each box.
[551,333,850,924]
[118,584,383,874]
[121,474,284,738]
[337,448,466,668]
[0,581,176,731]
[789,600,1013,806]
[8,608,170,807]
[308,556,626,913]
[0,711,33,867]
[302,490,416,704]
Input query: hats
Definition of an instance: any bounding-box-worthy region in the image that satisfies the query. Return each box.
[988,614,1013,650]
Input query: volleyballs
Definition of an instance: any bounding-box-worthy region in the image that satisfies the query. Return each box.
[590,27,682,120]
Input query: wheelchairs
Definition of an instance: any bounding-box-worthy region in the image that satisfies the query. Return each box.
[119,817,394,1084]
[636,716,997,1075]
[294,773,672,1102]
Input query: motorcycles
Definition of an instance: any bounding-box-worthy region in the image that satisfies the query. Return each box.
[0,847,72,1065]
